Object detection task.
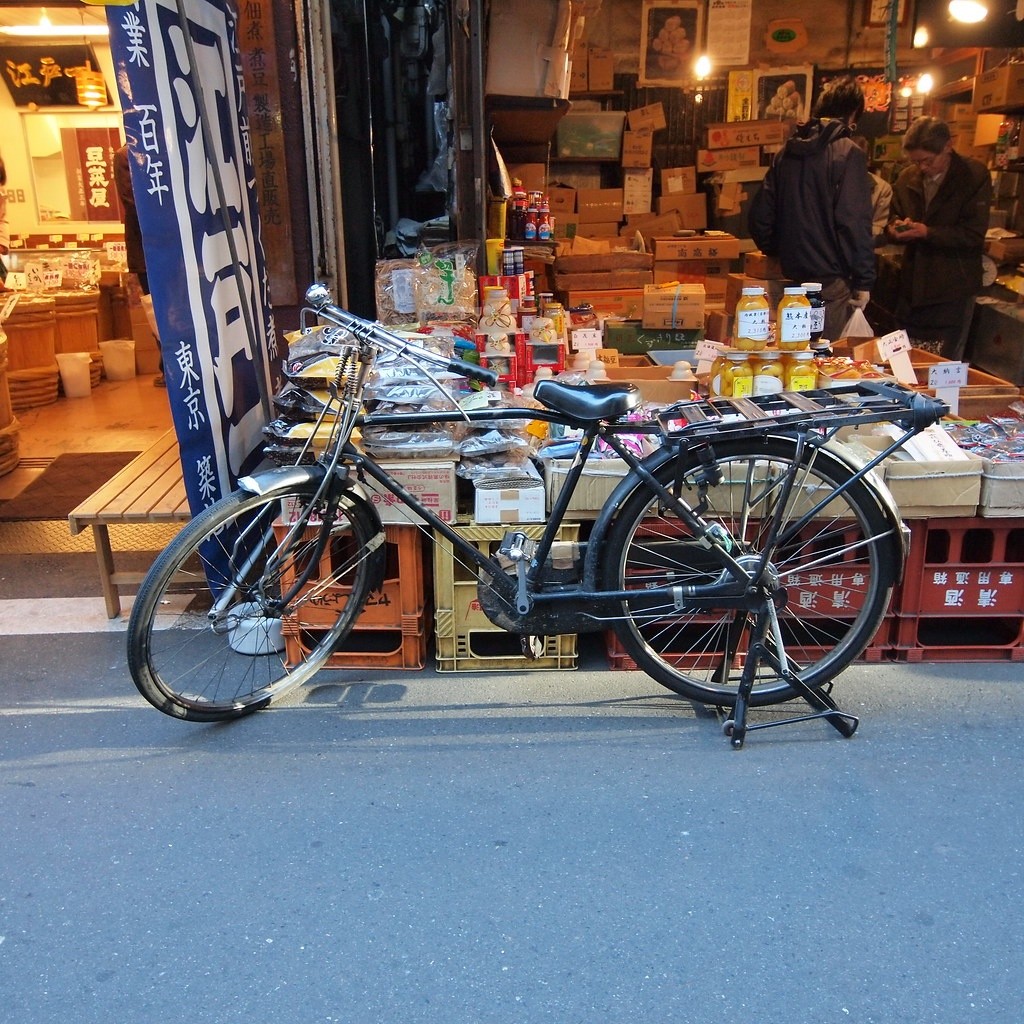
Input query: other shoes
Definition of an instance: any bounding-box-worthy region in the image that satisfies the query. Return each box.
[153,375,166,387]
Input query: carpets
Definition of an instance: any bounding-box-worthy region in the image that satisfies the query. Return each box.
[0,449,142,521]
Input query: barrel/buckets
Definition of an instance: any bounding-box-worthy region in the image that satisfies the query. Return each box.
[98,340,135,381]
[56,353,92,399]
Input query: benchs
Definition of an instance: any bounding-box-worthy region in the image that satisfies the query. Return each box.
[68,427,241,620]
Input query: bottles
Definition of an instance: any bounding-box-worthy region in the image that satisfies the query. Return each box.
[813,339,832,359]
[509,179,554,242]
[517,292,565,340]
[752,352,786,397]
[776,287,812,350]
[996,123,1009,171]
[784,352,819,392]
[733,287,770,351]
[1009,122,1021,162]
[720,353,752,399]
[483,286,502,309]
[486,238,525,276]
[802,282,826,343]
[709,350,726,397]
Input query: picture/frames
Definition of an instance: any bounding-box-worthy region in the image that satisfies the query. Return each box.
[638,0,703,88]
[272,48,1024,674]
[750,67,813,123]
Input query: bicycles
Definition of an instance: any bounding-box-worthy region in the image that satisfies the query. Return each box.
[127,283,953,748]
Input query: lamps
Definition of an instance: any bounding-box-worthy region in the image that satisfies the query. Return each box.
[75,9,108,106]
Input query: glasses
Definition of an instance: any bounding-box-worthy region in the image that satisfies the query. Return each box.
[909,151,938,165]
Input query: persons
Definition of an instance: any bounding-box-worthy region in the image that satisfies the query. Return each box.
[750,76,893,341]
[887,114,991,361]
[0,157,9,255]
[114,143,166,387]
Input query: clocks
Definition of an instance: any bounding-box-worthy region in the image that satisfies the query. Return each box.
[865,0,910,29]
